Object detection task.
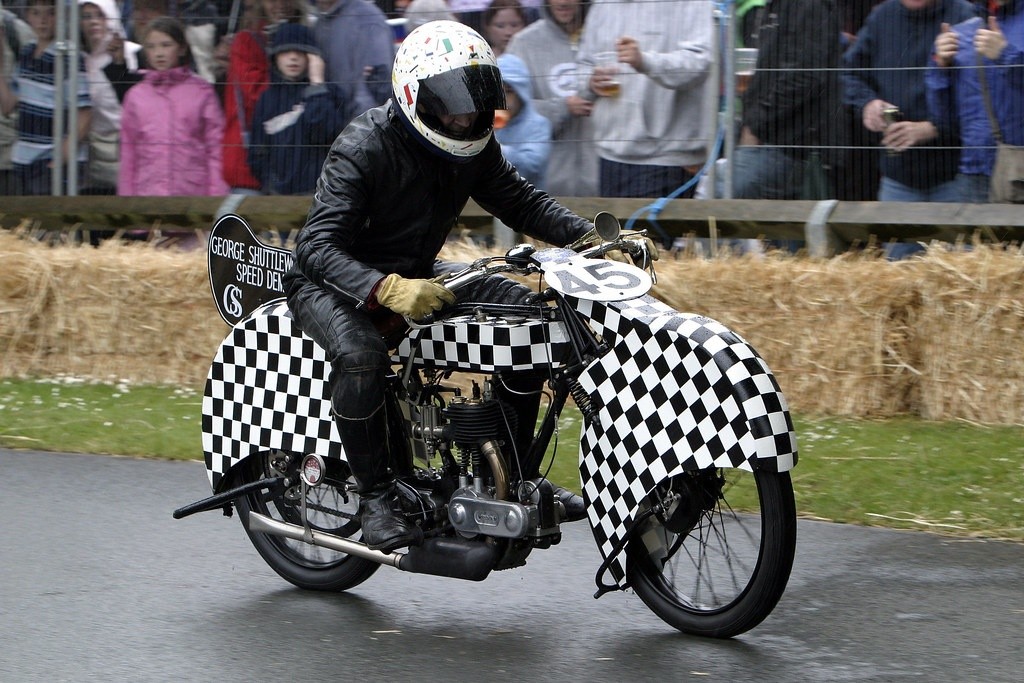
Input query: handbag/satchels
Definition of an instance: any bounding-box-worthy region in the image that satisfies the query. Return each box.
[989,142,1024,206]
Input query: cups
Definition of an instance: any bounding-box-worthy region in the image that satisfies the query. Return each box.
[593,51,622,94]
[732,47,758,92]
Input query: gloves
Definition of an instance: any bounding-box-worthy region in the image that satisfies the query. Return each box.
[593,227,659,271]
[376,273,459,325]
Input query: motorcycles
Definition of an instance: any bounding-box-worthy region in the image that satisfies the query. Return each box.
[171,212,799,640]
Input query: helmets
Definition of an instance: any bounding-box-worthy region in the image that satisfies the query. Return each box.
[391,21,507,158]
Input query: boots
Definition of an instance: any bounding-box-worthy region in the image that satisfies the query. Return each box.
[329,402,416,552]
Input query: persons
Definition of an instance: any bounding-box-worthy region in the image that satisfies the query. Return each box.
[0,0,1024,256]
[279,18,658,551]
